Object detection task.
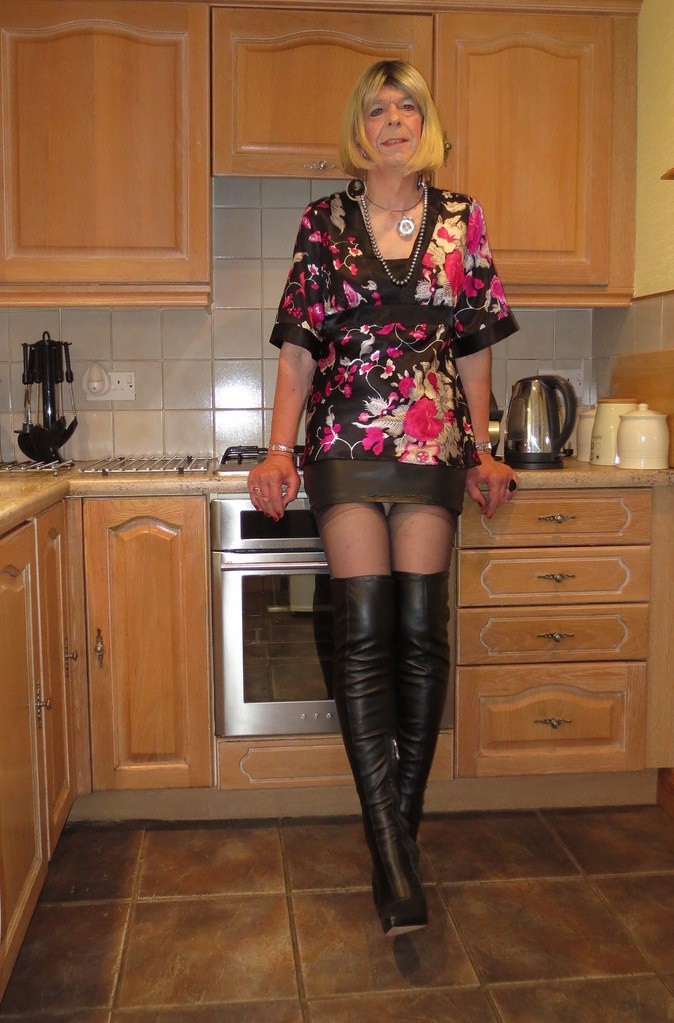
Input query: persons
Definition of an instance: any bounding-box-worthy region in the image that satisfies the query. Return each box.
[246,58,523,939]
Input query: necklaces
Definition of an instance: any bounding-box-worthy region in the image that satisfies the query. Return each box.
[362,184,424,236]
[360,179,429,286]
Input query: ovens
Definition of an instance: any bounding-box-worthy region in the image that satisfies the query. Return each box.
[210,492,457,738]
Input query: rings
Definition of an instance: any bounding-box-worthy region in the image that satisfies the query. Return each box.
[506,479,517,492]
[253,487,262,494]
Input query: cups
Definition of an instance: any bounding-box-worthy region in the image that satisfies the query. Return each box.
[616,402,670,469]
[590,397,640,466]
[577,409,597,462]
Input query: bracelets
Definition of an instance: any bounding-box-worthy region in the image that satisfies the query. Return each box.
[267,444,295,459]
[475,441,492,453]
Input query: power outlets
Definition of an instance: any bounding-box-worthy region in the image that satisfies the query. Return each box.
[86,371,136,401]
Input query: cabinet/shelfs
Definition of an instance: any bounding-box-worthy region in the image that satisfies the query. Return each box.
[209,0,434,184]
[1,520,53,1005]
[32,497,78,863]
[75,495,214,797]
[456,488,674,778]
[426,1,641,308]
[0,0,212,307]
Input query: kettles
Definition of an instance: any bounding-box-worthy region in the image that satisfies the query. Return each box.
[504,375,578,470]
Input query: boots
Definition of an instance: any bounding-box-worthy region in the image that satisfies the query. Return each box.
[331,571,448,939]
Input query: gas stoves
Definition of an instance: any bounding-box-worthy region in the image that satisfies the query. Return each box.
[217,445,305,476]
